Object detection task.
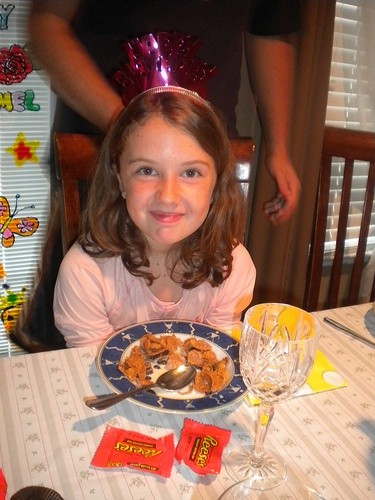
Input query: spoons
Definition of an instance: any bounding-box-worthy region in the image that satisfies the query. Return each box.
[83,365,197,411]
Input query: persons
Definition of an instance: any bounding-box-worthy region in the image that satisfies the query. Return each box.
[23,0,301,345]
[53,87,256,348]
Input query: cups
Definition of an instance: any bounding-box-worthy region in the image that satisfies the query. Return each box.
[218,477,329,500]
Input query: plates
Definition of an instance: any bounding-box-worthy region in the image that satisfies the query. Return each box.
[94,320,251,416]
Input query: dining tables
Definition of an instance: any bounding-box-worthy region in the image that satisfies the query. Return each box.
[0,303,375,500]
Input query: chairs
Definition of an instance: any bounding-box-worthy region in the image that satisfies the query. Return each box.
[53,132,255,258]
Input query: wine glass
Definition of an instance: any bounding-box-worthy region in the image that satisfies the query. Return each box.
[224,302,324,491]
